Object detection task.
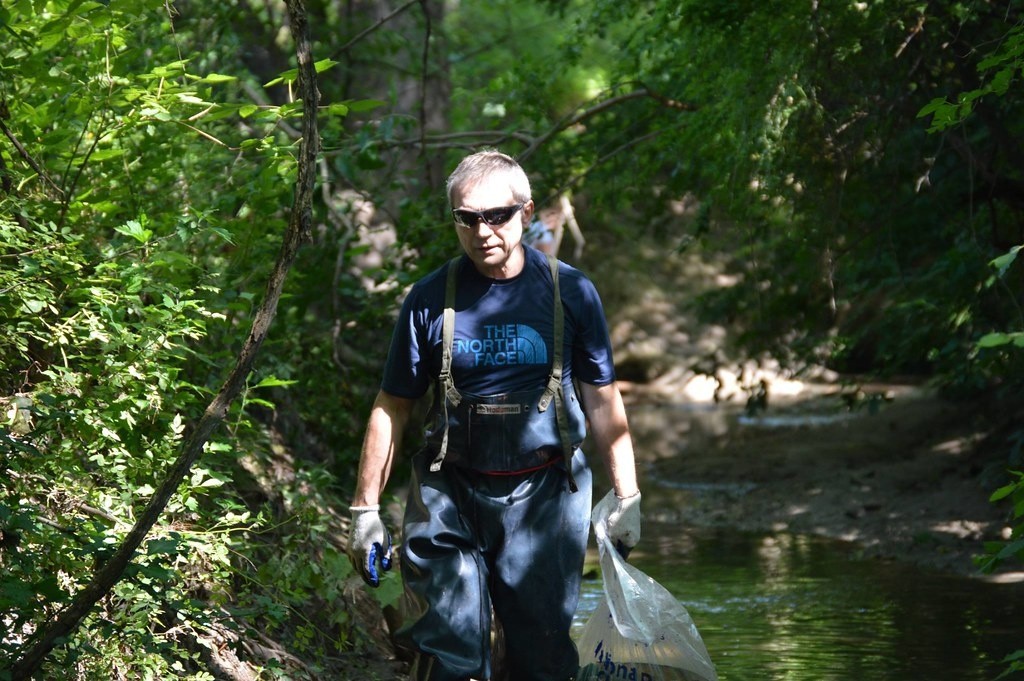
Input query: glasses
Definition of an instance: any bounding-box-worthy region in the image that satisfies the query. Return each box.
[451,203,523,228]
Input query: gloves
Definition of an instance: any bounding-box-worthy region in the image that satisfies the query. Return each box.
[345,505,392,588]
[606,490,642,561]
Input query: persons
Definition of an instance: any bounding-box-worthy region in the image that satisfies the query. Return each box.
[351,150,640,681]
